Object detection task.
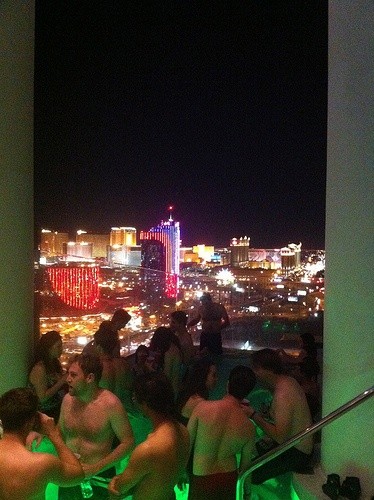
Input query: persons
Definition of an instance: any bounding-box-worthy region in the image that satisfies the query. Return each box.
[187,293,230,351]
[0,309,255,500]
[240,348,313,485]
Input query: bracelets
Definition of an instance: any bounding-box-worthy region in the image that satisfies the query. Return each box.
[250,411,256,419]
